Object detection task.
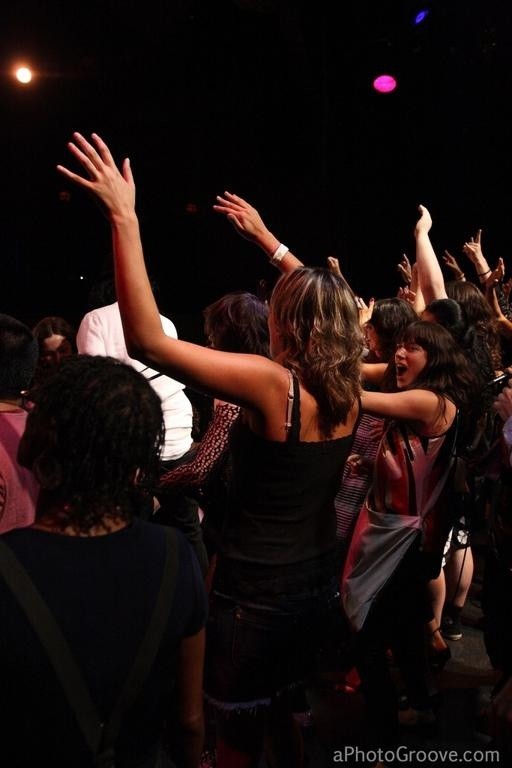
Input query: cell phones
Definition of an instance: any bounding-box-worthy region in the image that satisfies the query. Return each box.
[487,374,507,385]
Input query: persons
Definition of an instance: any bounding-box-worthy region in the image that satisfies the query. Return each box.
[0,202,512,767]
[53,128,367,766]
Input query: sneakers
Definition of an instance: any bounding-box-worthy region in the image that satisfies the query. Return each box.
[442,617,463,640]
[399,707,436,724]
